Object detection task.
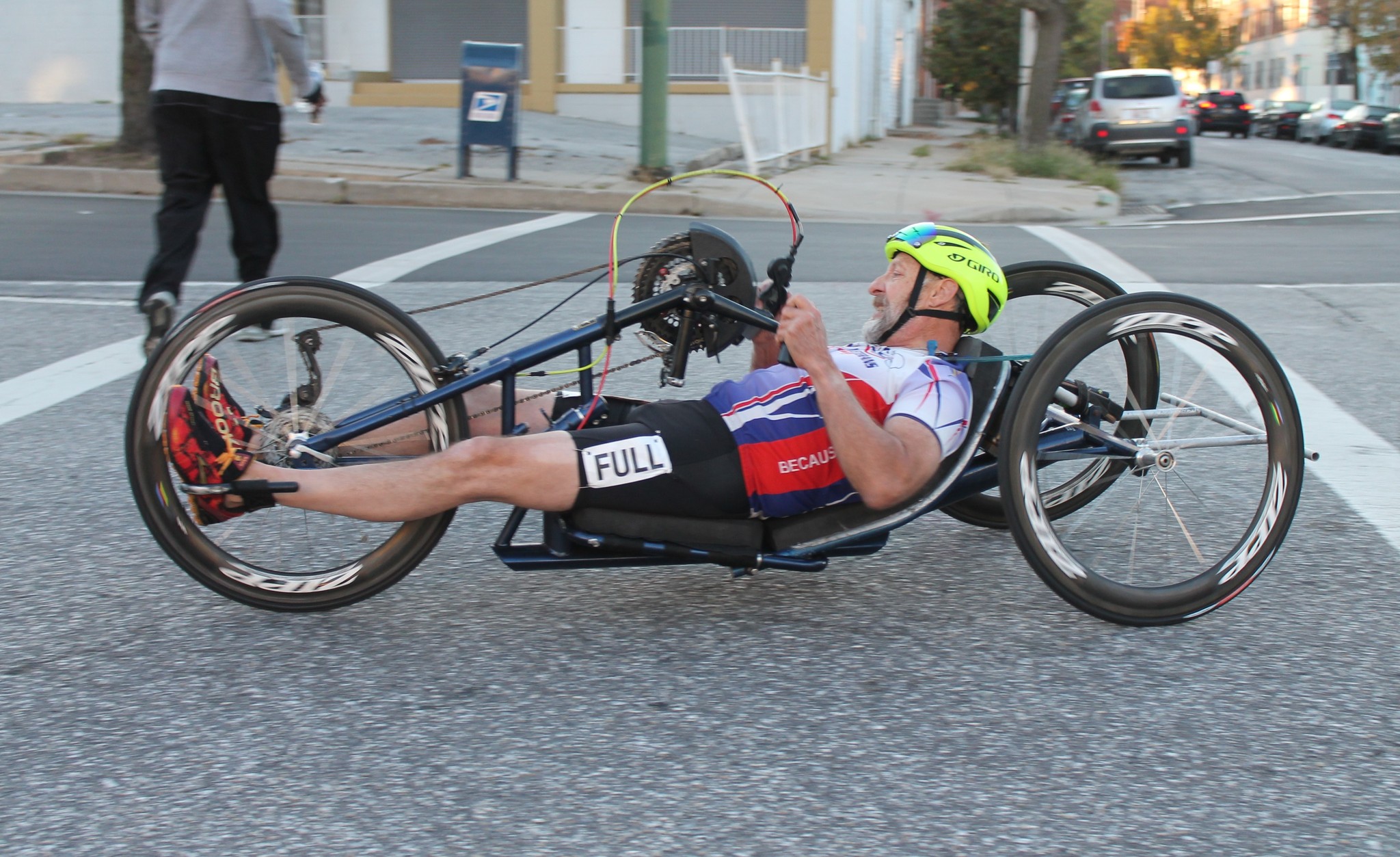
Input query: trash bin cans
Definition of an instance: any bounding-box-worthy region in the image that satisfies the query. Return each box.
[460,40,524,150]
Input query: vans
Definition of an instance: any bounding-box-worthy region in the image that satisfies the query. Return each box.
[1071,68,1193,168]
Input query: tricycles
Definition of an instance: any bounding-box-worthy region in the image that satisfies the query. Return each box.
[121,164,1320,627]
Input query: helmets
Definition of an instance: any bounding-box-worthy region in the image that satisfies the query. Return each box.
[885,223,1009,335]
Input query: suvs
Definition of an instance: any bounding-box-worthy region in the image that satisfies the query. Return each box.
[1188,92,1251,136]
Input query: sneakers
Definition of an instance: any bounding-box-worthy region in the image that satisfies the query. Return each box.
[194,355,264,451]
[162,385,244,525]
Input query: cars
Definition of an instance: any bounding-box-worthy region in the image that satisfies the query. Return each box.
[1049,77,1094,140]
[1247,99,1400,155]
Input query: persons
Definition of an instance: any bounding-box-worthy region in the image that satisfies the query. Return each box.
[163,222,1008,528]
[129,0,328,364]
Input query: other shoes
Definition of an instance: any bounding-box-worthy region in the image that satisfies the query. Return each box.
[141,290,176,364]
[236,317,291,340]
